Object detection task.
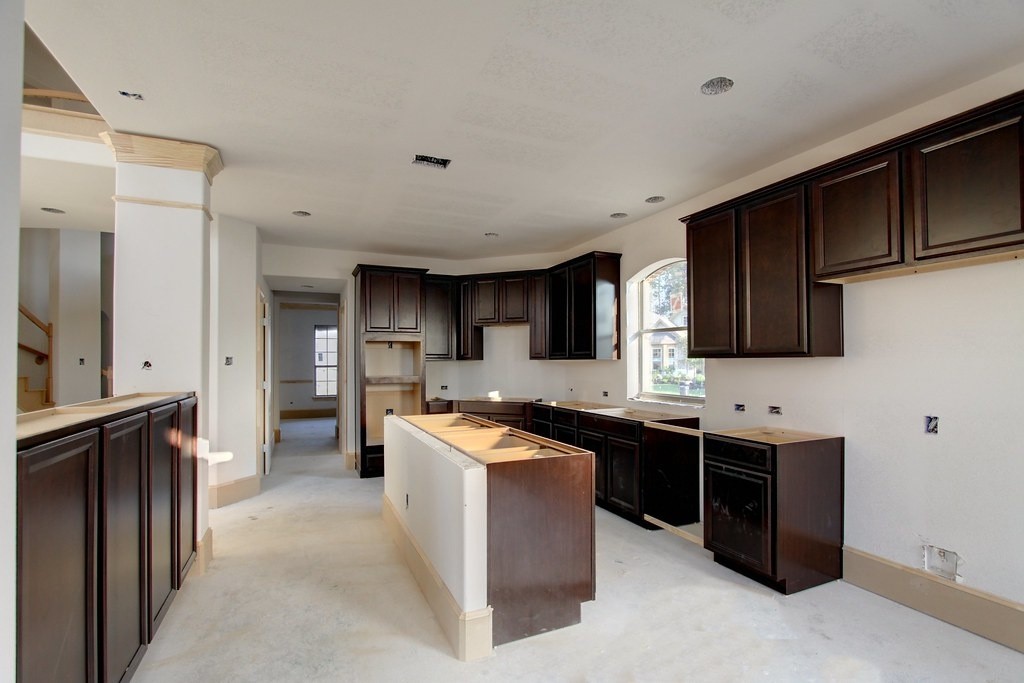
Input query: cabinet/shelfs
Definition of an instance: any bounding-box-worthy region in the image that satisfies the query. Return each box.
[702,425,845,595]
[809,151,906,286]
[424,273,457,361]
[577,410,700,531]
[686,182,843,358]
[470,270,529,326]
[907,89,1024,273]
[354,263,426,337]
[548,251,622,360]
[17,411,148,683]
[531,402,579,448]
[456,399,532,435]
[45,392,199,645]
